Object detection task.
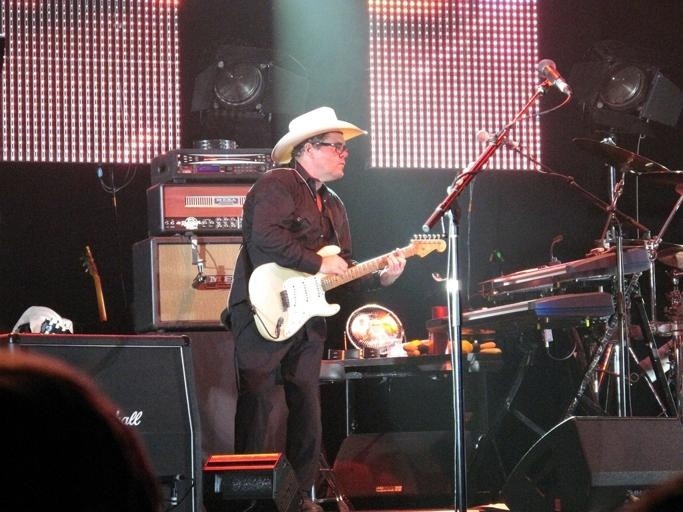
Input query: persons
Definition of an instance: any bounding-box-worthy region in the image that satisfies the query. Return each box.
[0,347,164,511]
[219,104,408,512]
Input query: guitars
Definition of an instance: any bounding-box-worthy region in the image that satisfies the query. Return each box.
[248,234,446,342]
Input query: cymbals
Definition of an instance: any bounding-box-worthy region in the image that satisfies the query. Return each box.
[652,170,682,184]
[573,138,671,174]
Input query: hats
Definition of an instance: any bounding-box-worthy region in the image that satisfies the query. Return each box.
[270,107,369,166]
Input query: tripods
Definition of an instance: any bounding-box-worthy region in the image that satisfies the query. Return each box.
[563,272,683,419]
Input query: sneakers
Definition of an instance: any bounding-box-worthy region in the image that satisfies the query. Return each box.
[297,496,323,512]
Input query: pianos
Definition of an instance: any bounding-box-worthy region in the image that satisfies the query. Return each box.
[426,244,649,337]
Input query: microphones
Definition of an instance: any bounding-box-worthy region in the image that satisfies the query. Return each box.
[537,57,573,97]
[478,129,520,150]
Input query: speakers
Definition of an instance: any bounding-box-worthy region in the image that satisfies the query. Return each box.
[201,451,302,510]
[328,428,508,504]
[543,414,681,510]
[3,333,202,511]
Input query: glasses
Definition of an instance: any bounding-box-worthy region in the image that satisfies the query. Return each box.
[310,141,349,154]
